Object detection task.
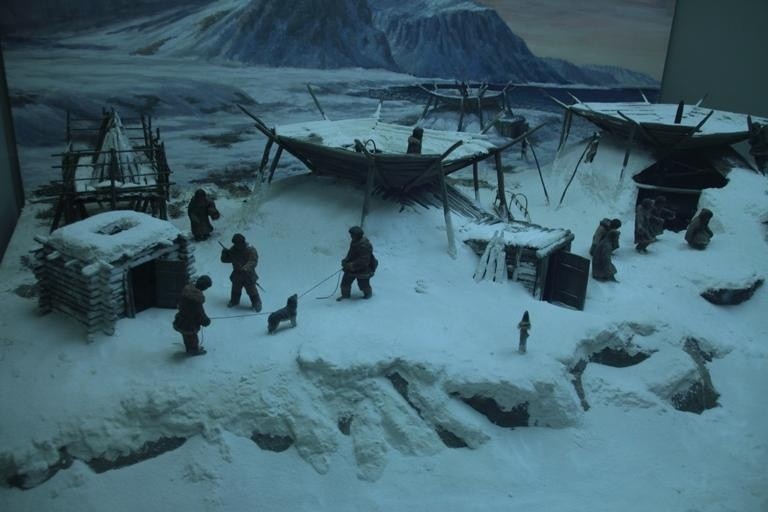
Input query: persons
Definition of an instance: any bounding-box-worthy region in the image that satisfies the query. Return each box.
[684,208,714,250]
[633,195,676,254]
[220,233,263,313]
[188,188,220,241]
[335,225,375,302]
[172,275,213,356]
[589,217,621,283]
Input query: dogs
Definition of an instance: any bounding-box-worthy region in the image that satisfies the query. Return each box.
[267,293,298,335]
